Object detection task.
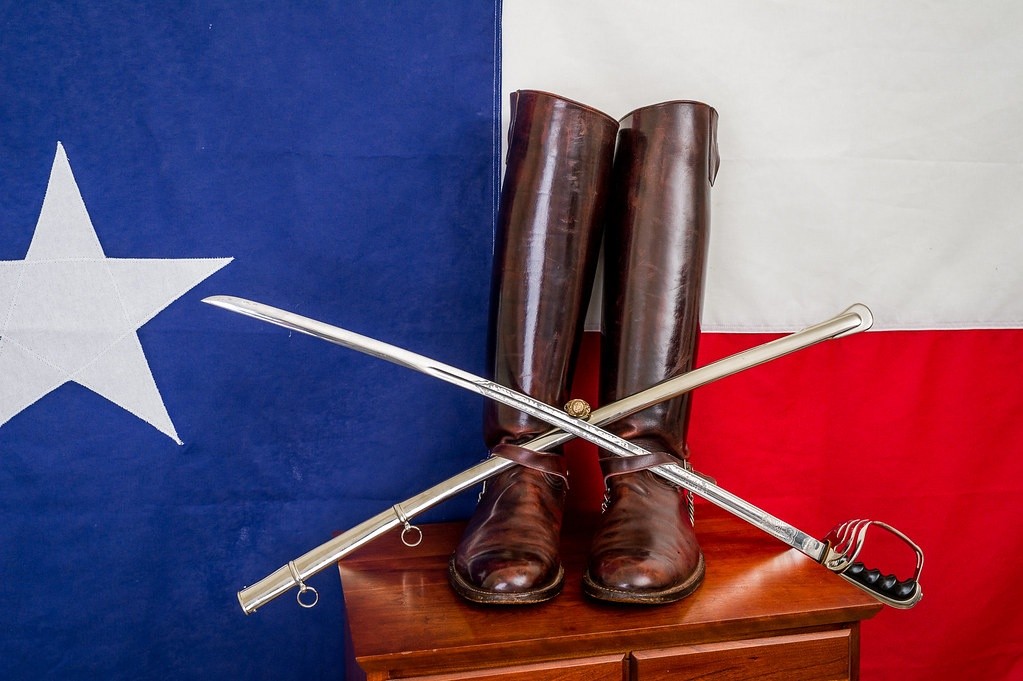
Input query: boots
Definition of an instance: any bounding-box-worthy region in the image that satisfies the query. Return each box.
[450,90,618,605]
[581,100,719,606]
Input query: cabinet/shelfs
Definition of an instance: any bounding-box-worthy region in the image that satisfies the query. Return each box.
[333,503,886,681]
